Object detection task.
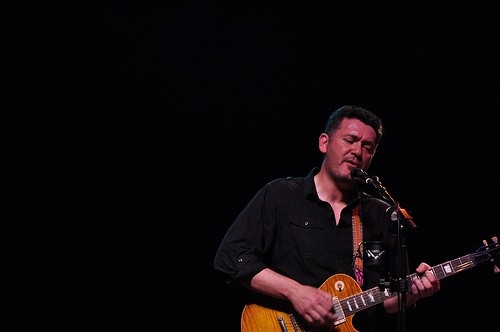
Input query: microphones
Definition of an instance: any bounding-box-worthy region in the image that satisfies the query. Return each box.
[350,168,378,187]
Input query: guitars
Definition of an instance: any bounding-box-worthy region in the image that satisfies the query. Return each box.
[240,236,500,332]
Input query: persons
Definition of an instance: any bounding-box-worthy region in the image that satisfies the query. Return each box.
[213,107,442,332]
